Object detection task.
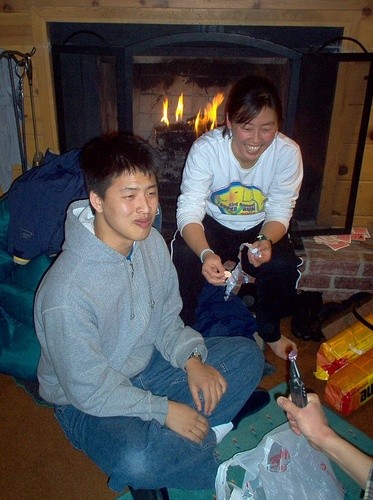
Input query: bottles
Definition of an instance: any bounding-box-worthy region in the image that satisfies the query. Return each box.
[288,353,308,410]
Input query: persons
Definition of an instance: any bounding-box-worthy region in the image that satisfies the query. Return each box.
[169,74,304,361]
[33,129,265,493]
[276,392,373,500]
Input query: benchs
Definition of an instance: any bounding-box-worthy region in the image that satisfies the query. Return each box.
[217,232,373,340]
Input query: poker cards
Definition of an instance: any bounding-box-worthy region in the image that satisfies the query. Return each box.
[313,224,372,253]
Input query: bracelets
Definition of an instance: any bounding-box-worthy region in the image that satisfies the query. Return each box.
[200,248,214,263]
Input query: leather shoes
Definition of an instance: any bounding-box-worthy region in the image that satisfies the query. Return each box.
[127,483,170,500]
[230,387,271,431]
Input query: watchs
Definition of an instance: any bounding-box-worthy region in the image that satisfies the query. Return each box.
[254,234,273,246]
[188,348,203,362]
